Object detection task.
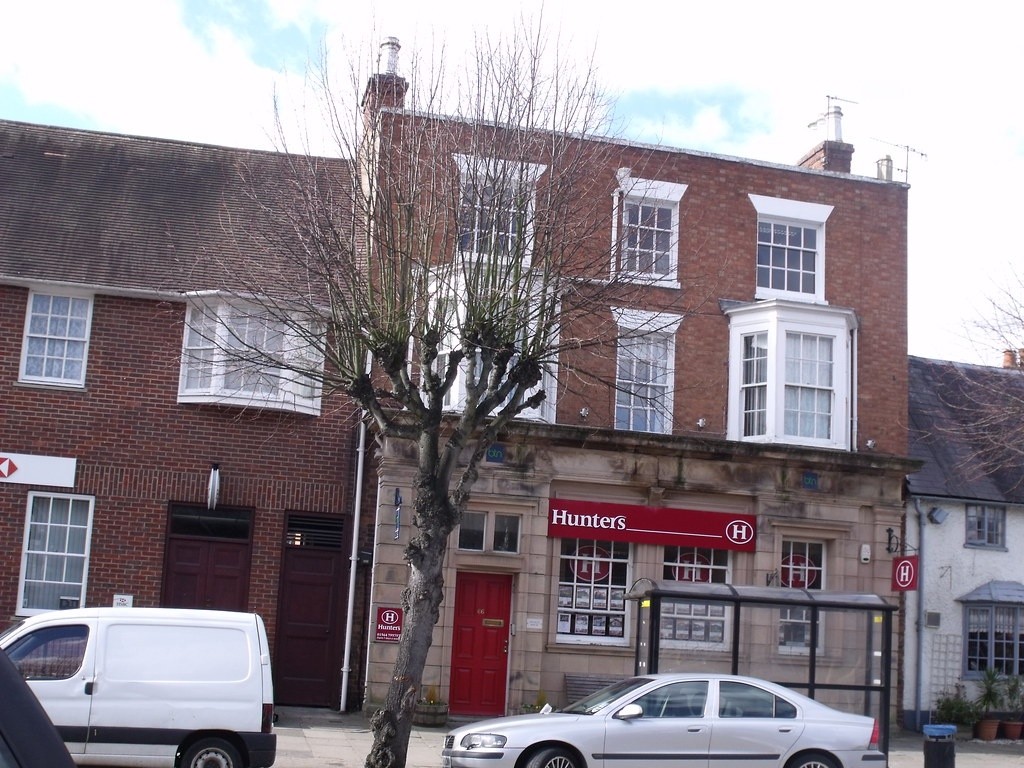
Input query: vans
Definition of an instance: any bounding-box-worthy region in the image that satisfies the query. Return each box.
[0,606,276,768]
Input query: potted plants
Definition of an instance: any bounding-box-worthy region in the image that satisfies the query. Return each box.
[931,692,981,740]
[412,682,449,728]
[974,667,1007,742]
[519,690,558,715]
[1002,673,1024,740]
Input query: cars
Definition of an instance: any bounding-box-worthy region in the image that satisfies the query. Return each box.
[441,672,886,768]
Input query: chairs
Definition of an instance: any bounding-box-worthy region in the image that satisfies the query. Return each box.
[673,693,692,717]
[691,692,706,717]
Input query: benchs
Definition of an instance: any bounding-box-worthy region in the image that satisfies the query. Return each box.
[564,672,633,705]
[741,699,795,718]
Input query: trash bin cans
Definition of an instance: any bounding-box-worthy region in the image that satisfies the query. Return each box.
[922,723,956,768]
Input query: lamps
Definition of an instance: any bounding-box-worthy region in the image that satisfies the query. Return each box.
[696,418,706,428]
[580,407,589,418]
[867,439,879,449]
[371,551,372,571]
[929,507,949,524]
